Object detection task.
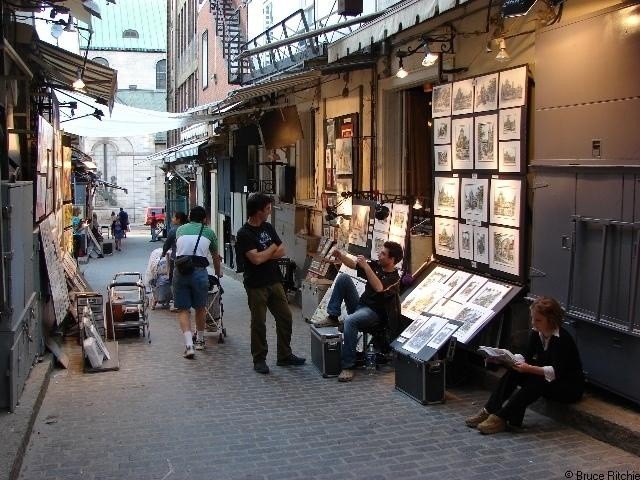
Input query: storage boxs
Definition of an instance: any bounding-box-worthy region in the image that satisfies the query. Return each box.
[310,324,341,378]
[299,277,333,321]
[392,348,446,405]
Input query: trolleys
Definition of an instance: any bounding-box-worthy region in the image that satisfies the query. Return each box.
[150,252,174,311]
[107,271,153,346]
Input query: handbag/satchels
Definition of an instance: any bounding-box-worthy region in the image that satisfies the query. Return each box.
[175,256,194,275]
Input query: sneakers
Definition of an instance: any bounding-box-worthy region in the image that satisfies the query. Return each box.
[277,353,304,366]
[183,345,195,359]
[254,358,269,373]
[193,334,205,350]
[466,408,489,427]
[478,414,505,434]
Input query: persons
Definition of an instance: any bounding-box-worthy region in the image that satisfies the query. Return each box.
[161,211,188,311]
[171,205,224,359]
[149,211,157,242]
[465,294,586,433]
[314,240,404,381]
[236,191,307,373]
[110,207,129,251]
[72,206,82,266]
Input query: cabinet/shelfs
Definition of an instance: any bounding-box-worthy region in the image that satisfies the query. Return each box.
[76,294,105,347]
[0,180,41,416]
[271,202,320,288]
[529,159,640,409]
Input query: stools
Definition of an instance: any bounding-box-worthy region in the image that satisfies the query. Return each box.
[337,320,379,375]
[101,226,109,240]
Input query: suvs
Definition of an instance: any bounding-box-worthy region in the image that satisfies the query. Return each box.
[144,206,166,228]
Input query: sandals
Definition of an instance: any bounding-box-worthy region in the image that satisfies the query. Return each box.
[314,316,339,327]
[338,369,353,381]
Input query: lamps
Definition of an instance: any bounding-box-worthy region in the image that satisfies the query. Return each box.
[374,197,410,221]
[62,13,76,32]
[413,188,430,210]
[493,39,511,61]
[325,190,368,221]
[421,44,439,67]
[71,69,86,90]
[395,58,410,79]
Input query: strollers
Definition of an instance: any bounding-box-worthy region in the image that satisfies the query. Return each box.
[204,275,229,345]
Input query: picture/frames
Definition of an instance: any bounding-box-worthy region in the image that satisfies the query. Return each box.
[324,225,336,238]
